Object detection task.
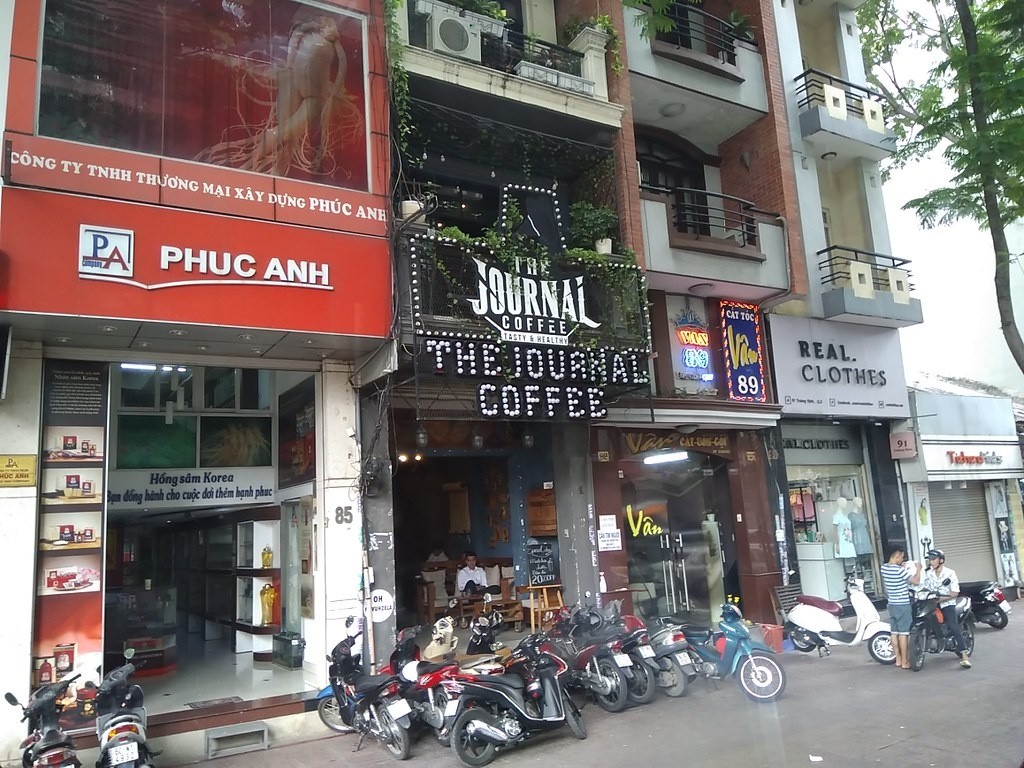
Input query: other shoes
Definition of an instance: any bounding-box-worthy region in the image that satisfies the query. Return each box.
[461,595,469,604]
[960,658,971,668]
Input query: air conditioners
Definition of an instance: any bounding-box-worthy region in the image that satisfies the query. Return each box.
[426,10,481,64]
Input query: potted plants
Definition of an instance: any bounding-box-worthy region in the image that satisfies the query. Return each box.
[415,0,515,37]
[567,200,618,255]
[589,13,624,79]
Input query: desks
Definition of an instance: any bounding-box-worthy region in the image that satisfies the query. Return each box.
[447,596,523,633]
[527,584,564,634]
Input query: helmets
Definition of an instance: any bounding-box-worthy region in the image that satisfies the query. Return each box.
[527,678,544,704]
[927,549,945,560]
[399,661,420,683]
[572,609,604,635]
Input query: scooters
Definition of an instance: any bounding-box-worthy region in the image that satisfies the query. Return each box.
[785,559,898,665]
[957,579,1013,630]
[81,648,165,768]
[4,671,85,768]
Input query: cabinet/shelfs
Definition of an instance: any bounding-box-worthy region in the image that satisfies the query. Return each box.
[146,505,285,663]
[525,488,558,536]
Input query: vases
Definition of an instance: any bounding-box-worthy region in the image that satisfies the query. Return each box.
[401,200,426,223]
[557,71,595,96]
[514,60,558,86]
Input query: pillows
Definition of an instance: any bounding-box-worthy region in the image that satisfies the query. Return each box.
[420,568,450,607]
[502,567,516,600]
[484,564,503,606]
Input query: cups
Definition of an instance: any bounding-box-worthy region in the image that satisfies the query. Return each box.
[145,579,151,590]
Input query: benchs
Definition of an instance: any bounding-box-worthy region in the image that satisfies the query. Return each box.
[415,557,514,628]
[516,584,562,626]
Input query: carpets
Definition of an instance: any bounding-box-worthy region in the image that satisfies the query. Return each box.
[188,696,244,709]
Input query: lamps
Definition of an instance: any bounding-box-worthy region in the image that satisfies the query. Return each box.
[944,480,967,491]
[674,424,698,434]
[687,284,715,295]
[821,152,837,161]
[414,423,534,449]
[166,366,193,425]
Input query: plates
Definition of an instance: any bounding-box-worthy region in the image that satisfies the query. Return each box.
[54,443,96,590]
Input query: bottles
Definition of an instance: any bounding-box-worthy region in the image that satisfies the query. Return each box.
[76,531,82,542]
[262,547,272,567]
[260,584,273,624]
[90,445,95,456]
[39,660,52,683]
[599,571,607,592]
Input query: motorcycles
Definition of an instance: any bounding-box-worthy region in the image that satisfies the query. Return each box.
[422,593,702,713]
[377,622,474,750]
[663,598,787,704]
[437,610,588,768]
[906,577,975,671]
[316,616,413,761]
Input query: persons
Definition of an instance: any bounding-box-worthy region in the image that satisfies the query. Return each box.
[425,545,448,562]
[917,550,971,668]
[458,552,501,595]
[881,544,922,669]
[832,496,875,558]
